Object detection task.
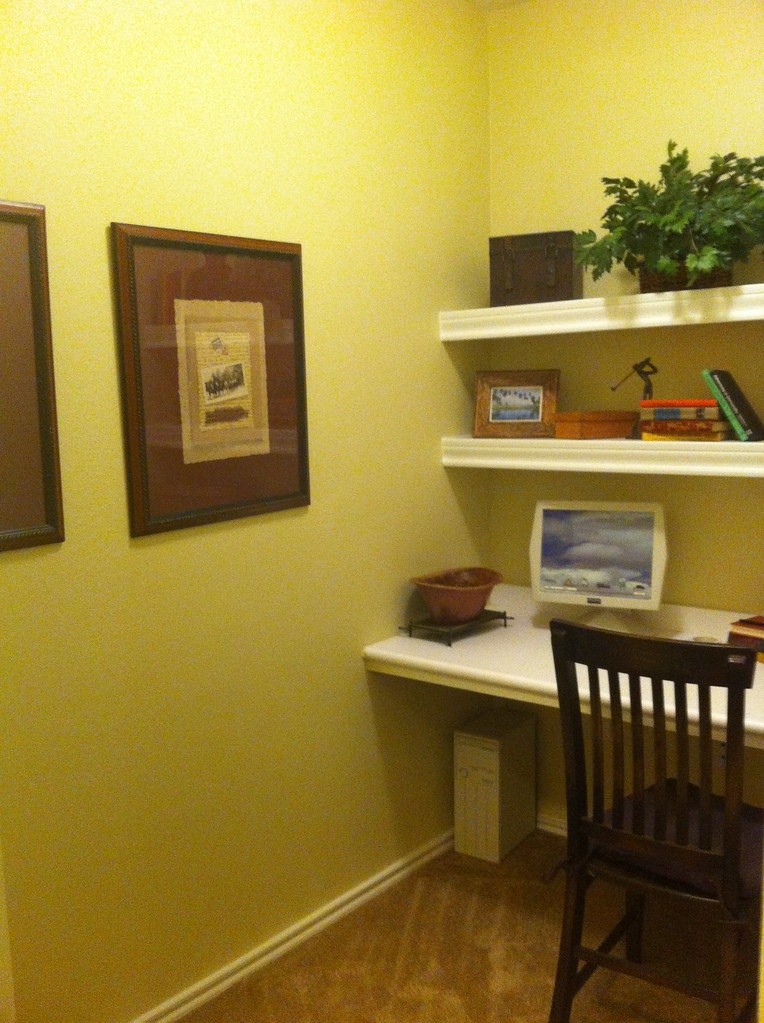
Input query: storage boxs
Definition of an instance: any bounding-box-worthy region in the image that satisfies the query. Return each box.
[550,411,639,438]
[490,231,584,307]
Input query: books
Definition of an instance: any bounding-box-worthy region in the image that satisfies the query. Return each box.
[731,615,764,664]
[640,399,735,441]
[700,368,764,441]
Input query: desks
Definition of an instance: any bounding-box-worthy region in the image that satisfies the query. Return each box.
[362,583,764,750]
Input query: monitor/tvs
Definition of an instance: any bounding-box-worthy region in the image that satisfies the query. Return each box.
[529,500,669,611]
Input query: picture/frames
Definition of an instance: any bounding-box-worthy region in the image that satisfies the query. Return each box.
[475,368,562,438]
[0,200,66,553]
[110,221,311,536]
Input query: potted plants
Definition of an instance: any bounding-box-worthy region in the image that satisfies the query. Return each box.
[573,139,764,293]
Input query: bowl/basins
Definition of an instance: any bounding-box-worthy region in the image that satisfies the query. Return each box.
[408,566,503,621]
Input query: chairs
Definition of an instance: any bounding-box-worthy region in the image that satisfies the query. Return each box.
[549,618,764,1023]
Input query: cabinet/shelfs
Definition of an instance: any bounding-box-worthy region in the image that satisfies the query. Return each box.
[439,281,764,480]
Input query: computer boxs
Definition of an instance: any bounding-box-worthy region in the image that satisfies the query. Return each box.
[451,706,537,862]
